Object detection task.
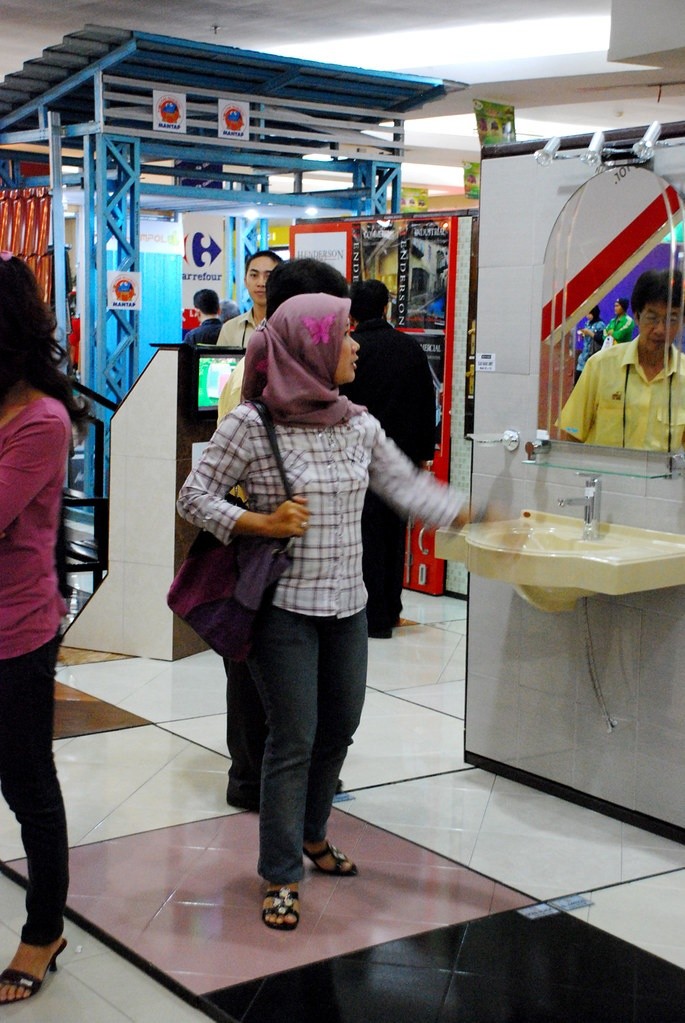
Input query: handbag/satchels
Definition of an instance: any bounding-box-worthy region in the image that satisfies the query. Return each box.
[167,492,294,661]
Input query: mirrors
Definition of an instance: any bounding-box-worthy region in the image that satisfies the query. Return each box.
[537,166,685,454]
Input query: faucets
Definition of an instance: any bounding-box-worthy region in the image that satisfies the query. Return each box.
[556,470,602,540]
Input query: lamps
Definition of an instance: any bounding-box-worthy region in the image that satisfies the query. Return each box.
[534,136,561,166]
[633,121,661,159]
[580,132,605,166]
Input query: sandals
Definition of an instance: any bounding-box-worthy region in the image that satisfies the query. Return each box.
[302,841,358,877]
[261,884,301,931]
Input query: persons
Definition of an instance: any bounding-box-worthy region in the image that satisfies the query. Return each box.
[221,255,344,813]
[604,295,632,345]
[337,278,438,640]
[0,255,78,1011]
[572,299,604,383]
[554,267,685,452]
[182,248,284,345]
[171,292,486,931]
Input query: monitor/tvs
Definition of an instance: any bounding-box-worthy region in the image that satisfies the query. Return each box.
[178,344,246,419]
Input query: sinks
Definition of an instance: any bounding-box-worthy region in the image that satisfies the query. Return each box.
[433,509,685,614]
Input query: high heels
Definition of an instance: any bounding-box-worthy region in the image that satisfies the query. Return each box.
[0,938,69,1004]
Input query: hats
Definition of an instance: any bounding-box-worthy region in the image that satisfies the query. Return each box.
[615,298,630,313]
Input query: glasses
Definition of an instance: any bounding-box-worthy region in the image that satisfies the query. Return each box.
[637,311,679,329]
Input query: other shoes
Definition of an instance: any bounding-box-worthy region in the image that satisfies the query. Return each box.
[227,789,259,814]
